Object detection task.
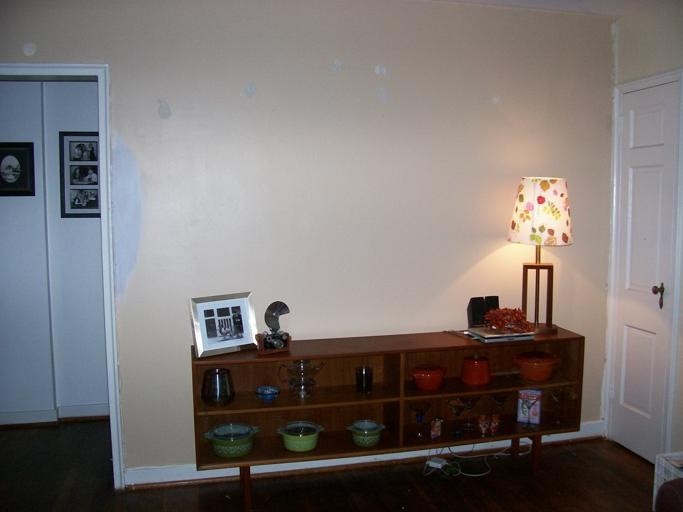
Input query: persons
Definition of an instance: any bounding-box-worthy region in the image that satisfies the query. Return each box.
[231,310,243,339]
[69,167,82,184]
[85,167,96,186]
[84,191,96,208]
[71,190,85,208]
[73,142,96,161]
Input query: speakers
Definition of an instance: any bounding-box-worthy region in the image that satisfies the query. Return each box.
[485,296,499,314]
[467,297,484,327]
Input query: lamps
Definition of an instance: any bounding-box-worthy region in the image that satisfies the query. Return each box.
[509,175,574,332]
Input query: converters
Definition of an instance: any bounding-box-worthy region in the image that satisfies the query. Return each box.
[426,456,447,469]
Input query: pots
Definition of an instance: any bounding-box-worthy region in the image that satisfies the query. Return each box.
[408,365,448,393]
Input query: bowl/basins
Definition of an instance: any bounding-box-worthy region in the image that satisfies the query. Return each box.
[273,419,324,452]
[253,385,281,400]
[203,423,263,458]
[510,345,561,384]
[345,419,385,448]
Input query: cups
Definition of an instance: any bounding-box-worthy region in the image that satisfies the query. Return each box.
[355,367,372,394]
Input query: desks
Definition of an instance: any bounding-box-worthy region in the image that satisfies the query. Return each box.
[650,451,681,512]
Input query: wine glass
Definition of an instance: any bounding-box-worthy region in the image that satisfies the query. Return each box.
[445,396,480,438]
[488,394,513,431]
[407,401,431,440]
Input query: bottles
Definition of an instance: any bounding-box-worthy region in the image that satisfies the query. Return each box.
[461,355,490,389]
[200,368,234,407]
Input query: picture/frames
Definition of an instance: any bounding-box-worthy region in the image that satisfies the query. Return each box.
[0,140,37,196]
[188,289,260,361]
[56,130,100,219]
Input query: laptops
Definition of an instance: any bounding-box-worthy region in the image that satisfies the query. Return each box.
[467,328,535,343]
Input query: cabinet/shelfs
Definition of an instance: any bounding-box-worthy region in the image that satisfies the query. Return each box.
[184,328,585,511]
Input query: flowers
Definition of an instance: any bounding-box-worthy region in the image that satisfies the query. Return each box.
[482,306,536,333]
